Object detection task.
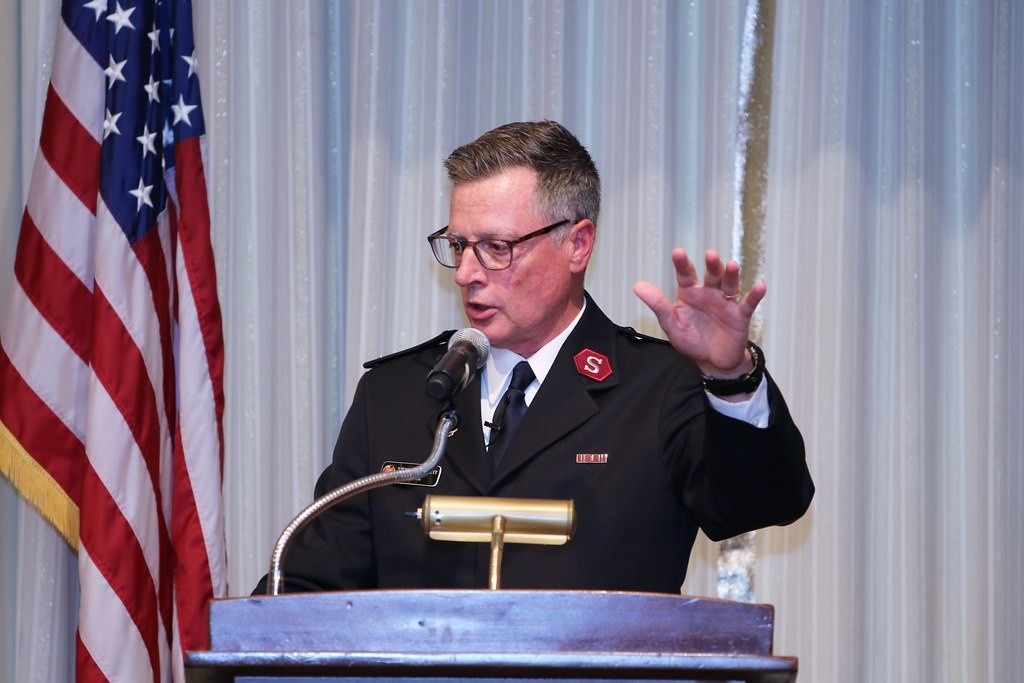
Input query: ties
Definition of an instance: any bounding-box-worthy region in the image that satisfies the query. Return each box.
[489,361,535,471]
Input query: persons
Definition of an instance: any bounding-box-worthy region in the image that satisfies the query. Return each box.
[248,120,816,595]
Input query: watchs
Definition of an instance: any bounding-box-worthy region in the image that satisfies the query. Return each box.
[699,339,766,396]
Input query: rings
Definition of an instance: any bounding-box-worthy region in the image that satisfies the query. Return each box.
[720,290,740,299]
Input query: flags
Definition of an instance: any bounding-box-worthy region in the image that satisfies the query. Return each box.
[0,1,230,682]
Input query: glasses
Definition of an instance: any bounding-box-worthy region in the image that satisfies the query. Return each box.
[427,217,571,270]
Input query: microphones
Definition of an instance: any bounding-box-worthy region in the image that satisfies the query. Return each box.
[422,327,491,402]
